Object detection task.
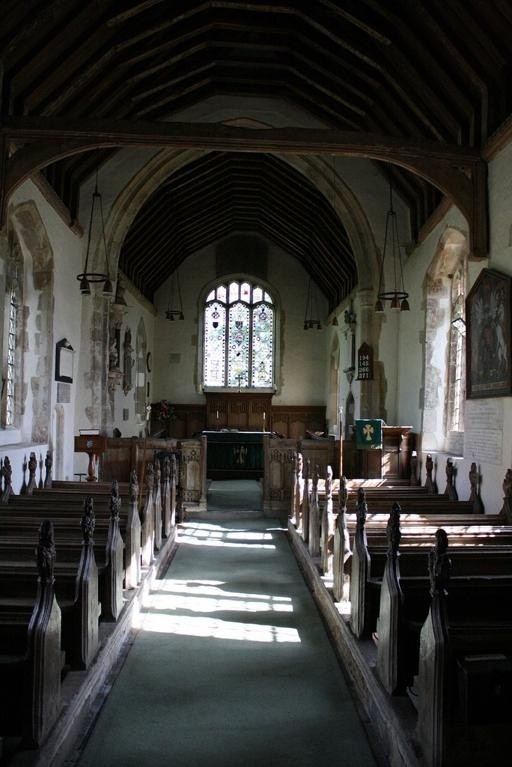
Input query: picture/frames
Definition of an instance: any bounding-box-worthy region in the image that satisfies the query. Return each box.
[465,268,512,399]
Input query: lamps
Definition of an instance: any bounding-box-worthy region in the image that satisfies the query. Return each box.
[77,148,113,296]
[303,277,322,330]
[375,163,410,312]
[165,268,184,321]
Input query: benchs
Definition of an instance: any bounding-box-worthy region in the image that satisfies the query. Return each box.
[0,497,102,671]
[408,527,512,765]
[24,452,171,538]
[375,501,512,696]
[303,457,458,541]
[350,488,512,639]
[296,453,436,528]
[289,451,418,513]
[44,450,176,528]
[1,479,125,621]
[0,462,155,567]
[1,469,141,589]
[1,519,62,747]
[0,456,163,550]
[309,463,485,557]
[320,465,512,575]
[333,475,512,602]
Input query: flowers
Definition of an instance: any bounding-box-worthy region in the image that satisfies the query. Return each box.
[156,400,178,436]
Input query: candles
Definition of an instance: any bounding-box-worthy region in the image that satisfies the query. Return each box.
[263,412,265,420]
[217,410,219,419]
